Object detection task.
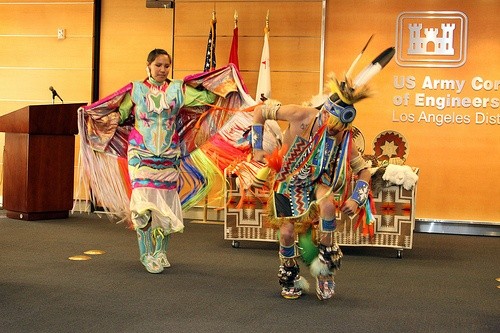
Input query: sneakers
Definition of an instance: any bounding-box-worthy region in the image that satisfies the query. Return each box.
[164,255,171,267]
[140,253,164,273]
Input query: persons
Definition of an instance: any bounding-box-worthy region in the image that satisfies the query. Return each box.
[251,88,371,301]
[78,49,234,276]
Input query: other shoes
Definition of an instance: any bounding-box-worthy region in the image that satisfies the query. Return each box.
[316,275,335,302]
[281,287,300,301]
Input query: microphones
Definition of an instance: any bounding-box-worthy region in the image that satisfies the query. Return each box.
[49,86,63,101]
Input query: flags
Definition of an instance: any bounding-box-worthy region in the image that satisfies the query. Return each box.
[203,2,217,72]
[255,10,272,101]
[228,9,240,72]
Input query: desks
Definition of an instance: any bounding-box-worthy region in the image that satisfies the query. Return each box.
[222,156,419,258]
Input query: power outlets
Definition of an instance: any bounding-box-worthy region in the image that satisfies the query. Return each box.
[57,29,66,39]
